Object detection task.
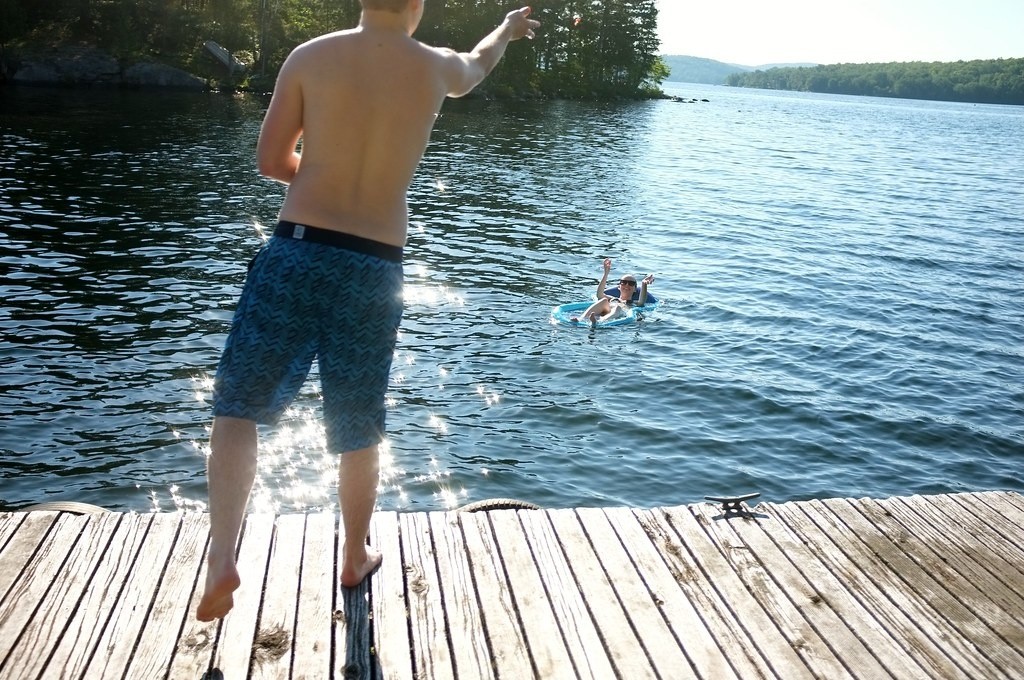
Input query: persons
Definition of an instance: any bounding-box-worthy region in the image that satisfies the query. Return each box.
[570,258,655,325]
[195,0,540,623]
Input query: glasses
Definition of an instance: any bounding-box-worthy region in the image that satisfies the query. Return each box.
[620,280,636,287]
